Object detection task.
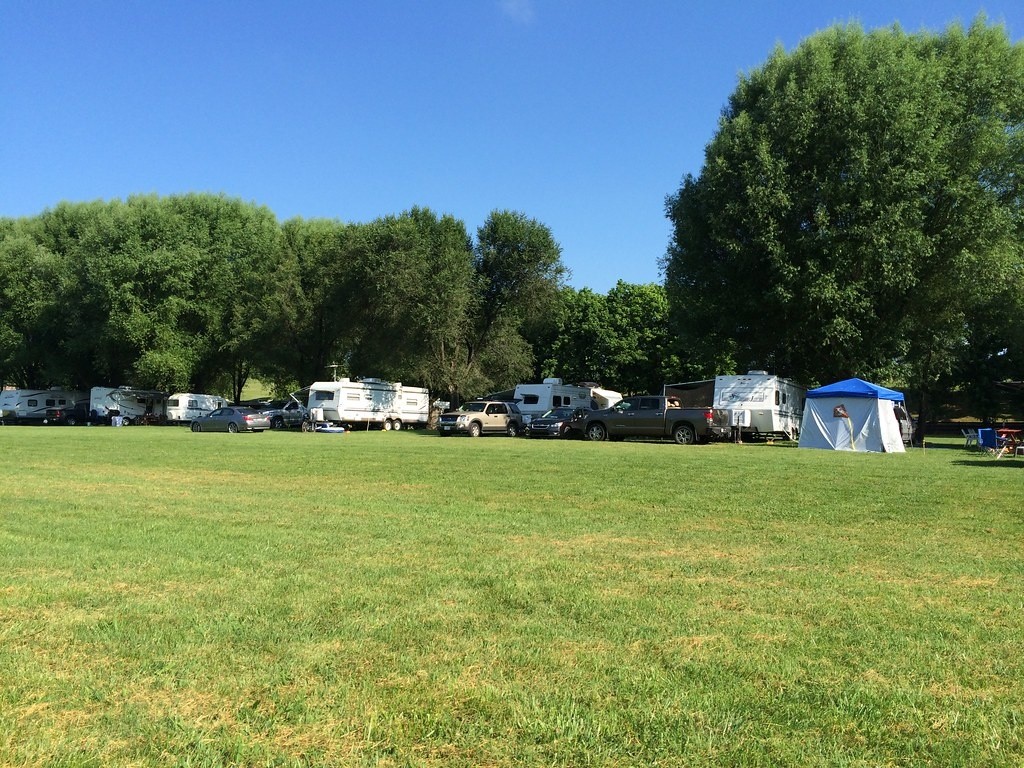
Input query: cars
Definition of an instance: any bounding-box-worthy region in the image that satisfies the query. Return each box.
[189,406,271,433]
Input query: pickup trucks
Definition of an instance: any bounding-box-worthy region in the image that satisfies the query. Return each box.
[582,393,730,445]
[45,401,90,426]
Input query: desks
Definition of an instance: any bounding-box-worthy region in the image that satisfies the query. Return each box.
[996,430,1021,445]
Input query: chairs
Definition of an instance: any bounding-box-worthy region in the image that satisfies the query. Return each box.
[962,428,1024,459]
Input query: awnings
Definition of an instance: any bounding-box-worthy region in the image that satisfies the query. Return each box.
[591,388,623,409]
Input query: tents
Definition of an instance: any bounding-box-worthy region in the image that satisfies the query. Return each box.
[797,377,914,453]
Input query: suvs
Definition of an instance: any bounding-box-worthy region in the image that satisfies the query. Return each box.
[255,400,307,429]
[523,404,593,438]
[436,398,524,437]
